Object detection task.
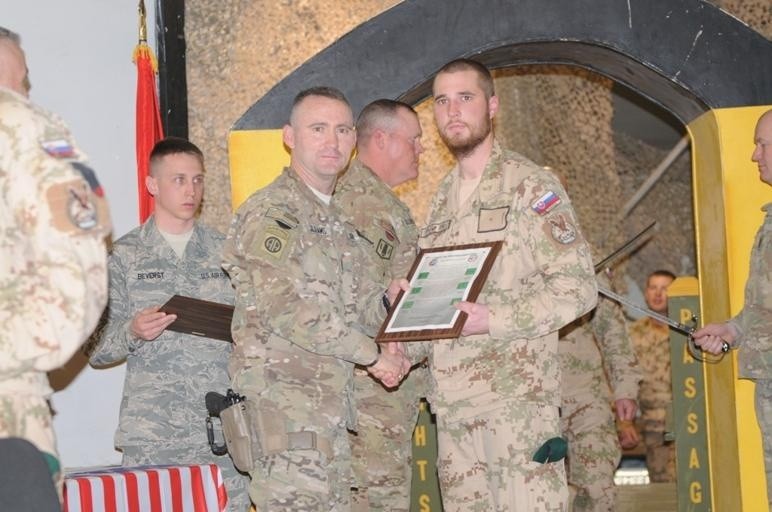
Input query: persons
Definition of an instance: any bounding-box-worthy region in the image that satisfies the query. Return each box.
[559,239,642,511]
[219,86,416,511]
[366,59,601,512]
[600,269,675,483]
[0,27,114,511]
[80,138,256,512]
[690,108,771,511]
[330,98,441,510]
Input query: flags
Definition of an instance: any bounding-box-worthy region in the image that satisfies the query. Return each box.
[131,41,168,227]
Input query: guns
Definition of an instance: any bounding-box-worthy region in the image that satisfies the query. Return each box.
[204,388,246,416]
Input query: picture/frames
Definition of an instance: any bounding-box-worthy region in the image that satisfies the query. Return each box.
[374,240,503,344]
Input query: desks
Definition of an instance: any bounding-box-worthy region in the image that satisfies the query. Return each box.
[54,463,227,512]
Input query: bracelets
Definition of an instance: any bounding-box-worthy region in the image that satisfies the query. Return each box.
[382,287,392,314]
[365,342,382,366]
